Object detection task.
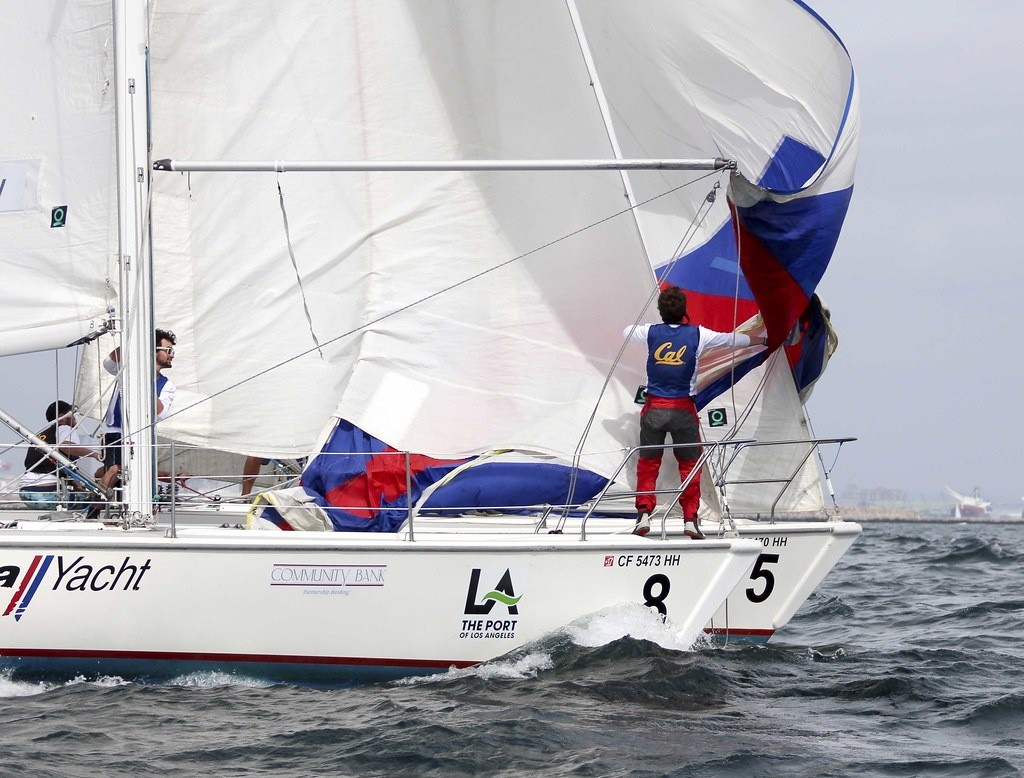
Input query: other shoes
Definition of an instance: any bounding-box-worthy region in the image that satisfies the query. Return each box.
[242,495,251,504]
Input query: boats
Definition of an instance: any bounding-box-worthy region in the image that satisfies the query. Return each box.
[947,484,991,521]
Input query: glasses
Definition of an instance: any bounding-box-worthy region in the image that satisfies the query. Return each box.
[157,347,175,355]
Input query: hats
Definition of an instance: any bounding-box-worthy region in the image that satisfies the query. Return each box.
[46,400,78,422]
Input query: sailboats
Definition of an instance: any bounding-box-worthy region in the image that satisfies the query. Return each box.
[0,3,866,671]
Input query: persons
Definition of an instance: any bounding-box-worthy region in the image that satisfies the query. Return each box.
[102,330,177,475]
[19,400,108,510]
[241,456,308,495]
[624,285,769,539]
[94,463,193,496]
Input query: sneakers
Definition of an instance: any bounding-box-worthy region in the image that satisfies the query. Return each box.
[631,512,652,536]
[684,522,706,540]
